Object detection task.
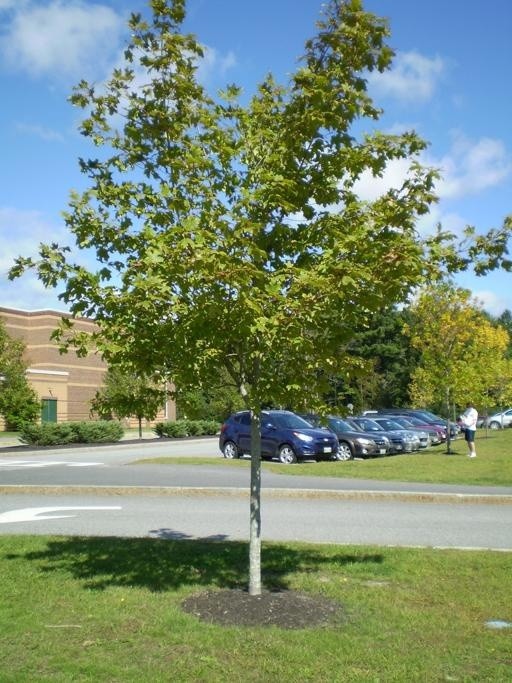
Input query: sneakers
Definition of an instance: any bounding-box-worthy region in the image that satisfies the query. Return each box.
[466,452,476,457]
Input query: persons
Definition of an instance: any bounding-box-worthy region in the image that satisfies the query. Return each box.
[457,402,479,458]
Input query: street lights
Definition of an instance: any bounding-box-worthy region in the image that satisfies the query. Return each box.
[154,369,171,422]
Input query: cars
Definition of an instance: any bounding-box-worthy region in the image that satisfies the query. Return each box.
[488,408,512,429]
[219,407,461,465]
[477,412,501,428]
